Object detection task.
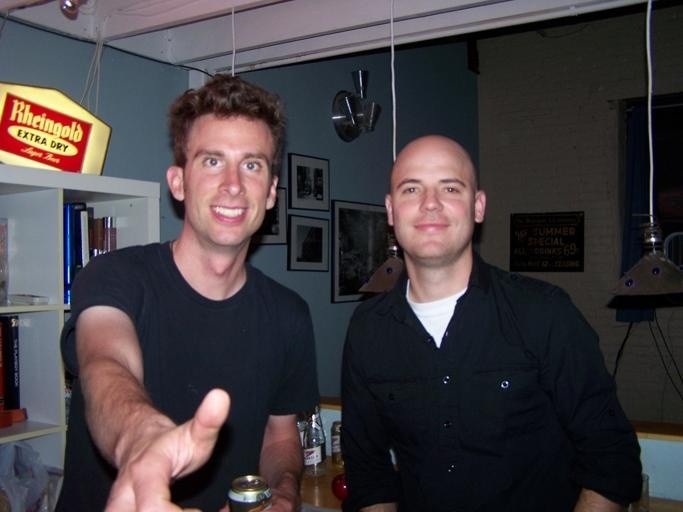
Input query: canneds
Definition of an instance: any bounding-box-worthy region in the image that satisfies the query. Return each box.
[228,475,272,512]
[332,422,345,469]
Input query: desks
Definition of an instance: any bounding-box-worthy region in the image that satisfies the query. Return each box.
[290,454,682,510]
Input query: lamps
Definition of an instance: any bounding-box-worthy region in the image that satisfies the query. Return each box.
[328,70,383,133]
[599,5,682,300]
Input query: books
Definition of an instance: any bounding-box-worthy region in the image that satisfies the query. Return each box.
[2,314,21,412]
[59,198,118,306]
[0,214,10,307]
[0,324,7,418]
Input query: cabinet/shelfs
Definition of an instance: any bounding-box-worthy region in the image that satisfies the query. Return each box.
[0,163,160,512]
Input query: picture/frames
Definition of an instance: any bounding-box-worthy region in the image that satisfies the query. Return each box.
[254,152,392,304]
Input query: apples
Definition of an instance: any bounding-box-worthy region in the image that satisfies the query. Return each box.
[332,473,349,501]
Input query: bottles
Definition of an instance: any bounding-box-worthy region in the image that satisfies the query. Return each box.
[303,403,327,478]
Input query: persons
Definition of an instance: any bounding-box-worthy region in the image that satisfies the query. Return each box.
[52,73,321,512]
[337,132,644,512]
